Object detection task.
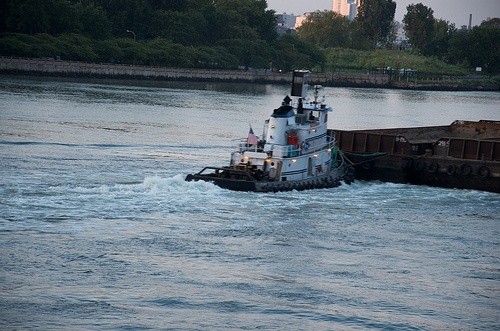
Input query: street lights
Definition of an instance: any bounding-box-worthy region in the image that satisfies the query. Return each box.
[127,29,136,66]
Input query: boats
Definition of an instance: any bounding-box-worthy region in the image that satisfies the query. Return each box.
[184,68,358,194]
